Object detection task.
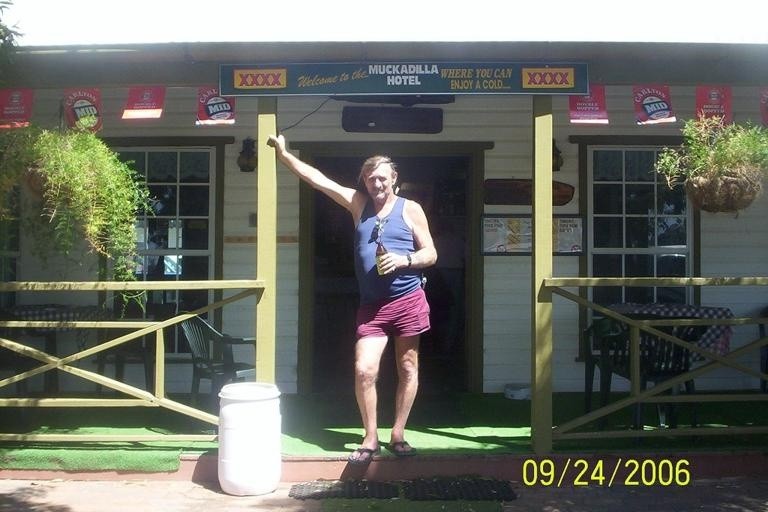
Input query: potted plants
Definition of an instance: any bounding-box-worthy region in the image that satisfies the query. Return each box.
[652,110,768,214]
[642,181,687,265]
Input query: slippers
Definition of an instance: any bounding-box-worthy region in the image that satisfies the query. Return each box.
[348,445,381,465]
[386,441,416,456]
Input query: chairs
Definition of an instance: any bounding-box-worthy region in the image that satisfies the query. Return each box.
[88,302,266,413]
[581,313,708,434]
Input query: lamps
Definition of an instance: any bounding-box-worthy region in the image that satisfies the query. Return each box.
[553,138,563,171]
[236,138,258,172]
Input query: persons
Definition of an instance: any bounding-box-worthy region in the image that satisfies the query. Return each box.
[267,132,438,463]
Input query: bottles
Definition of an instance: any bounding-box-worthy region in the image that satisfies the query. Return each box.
[376,242,392,280]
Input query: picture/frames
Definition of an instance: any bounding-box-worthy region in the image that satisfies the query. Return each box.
[478,213,589,257]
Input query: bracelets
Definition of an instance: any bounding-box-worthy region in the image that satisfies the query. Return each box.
[406,254,413,269]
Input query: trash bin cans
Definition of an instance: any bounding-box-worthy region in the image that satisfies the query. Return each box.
[216,381,282,496]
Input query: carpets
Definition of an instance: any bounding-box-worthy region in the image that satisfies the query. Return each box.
[2,380,768,472]
